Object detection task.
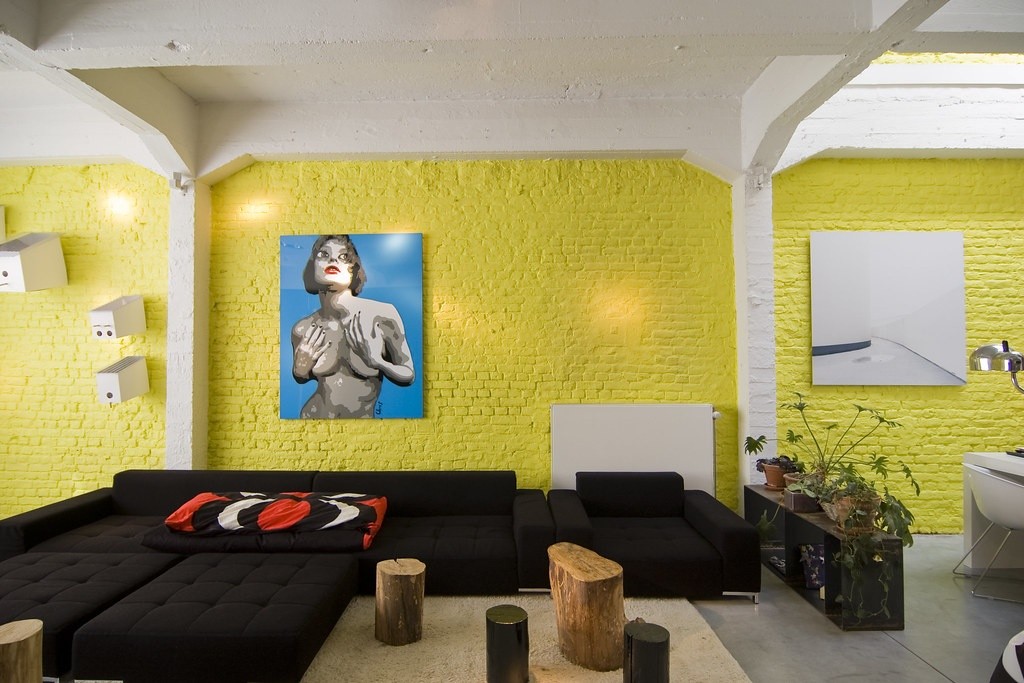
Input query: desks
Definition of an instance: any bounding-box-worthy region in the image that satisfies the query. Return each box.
[962,451,1024,579]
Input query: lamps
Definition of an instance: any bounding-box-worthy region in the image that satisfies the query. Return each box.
[971,340,1024,393]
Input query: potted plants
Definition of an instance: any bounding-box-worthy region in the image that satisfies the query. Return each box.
[742,388,922,548]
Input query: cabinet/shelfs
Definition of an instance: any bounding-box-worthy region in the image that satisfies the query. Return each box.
[743,483,906,631]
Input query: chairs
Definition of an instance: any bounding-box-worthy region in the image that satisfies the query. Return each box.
[952,462,1024,605]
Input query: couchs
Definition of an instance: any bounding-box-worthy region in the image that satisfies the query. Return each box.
[0,423,763,682]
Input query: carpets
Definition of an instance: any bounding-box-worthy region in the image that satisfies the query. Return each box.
[298,597,754,683]
